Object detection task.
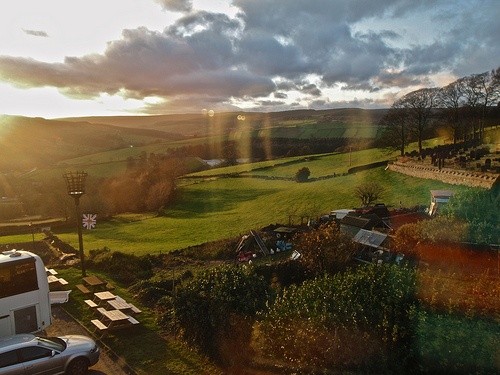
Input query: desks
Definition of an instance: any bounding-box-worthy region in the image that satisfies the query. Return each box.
[92,291,117,311]
[82,275,105,298]
[46,274,59,284]
[104,300,131,316]
[44,266,49,272]
[94,309,130,340]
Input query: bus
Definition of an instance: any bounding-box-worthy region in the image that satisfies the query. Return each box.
[1,249,52,334]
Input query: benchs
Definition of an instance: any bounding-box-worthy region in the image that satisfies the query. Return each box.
[98,306,107,316]
[58,278,70,286]
[49,268,57,275]
[127,302,141,316]
[126,315,140,326]
[90,320,108,332]
[83,299,96,309]
[76,283,90,295]
[102,278,114,290]
[115,294,126,303]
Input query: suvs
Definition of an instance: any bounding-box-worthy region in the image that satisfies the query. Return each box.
[0,334,99,375]
